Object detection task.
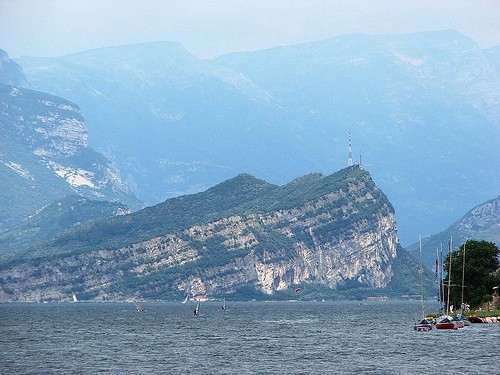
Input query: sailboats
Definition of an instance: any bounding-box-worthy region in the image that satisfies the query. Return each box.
[193,299,202,317]
[222,296,227,310]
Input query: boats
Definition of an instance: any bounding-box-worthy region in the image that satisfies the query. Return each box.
[414,233,470,334]
